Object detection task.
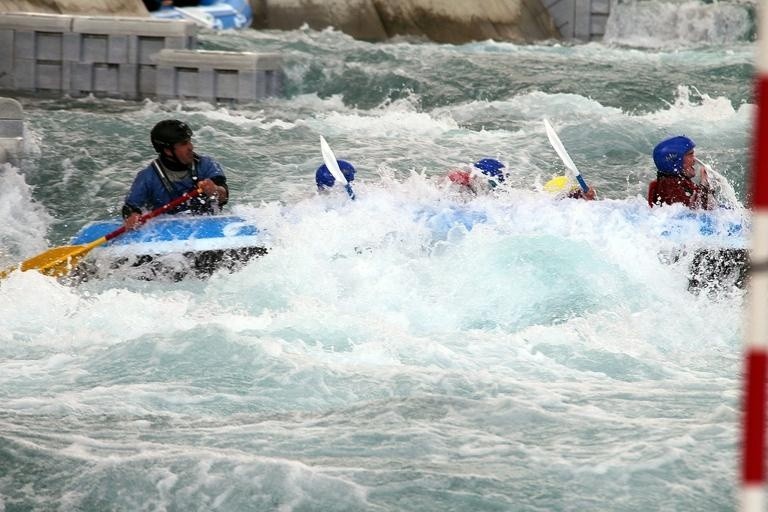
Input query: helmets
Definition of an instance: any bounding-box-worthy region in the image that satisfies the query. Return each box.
[652,138,696,176]
[150,120,193,153]
[315,161,356,192]
[476,158,509,184]
[543,175,581,199]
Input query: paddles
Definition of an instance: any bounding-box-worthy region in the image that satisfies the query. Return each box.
[320,134,355,199]
[542,118,589,192]
[0,188,202,280]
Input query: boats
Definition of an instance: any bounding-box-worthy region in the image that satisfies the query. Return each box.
[66,203,752,281]
[150,0,253,32]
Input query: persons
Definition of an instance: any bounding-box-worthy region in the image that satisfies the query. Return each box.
[315,161,356,198]
[445,172,480,196]
[543,175,595,201]
[647,136,726,211]
[122,119,229,232]
[472,160,509,192]
